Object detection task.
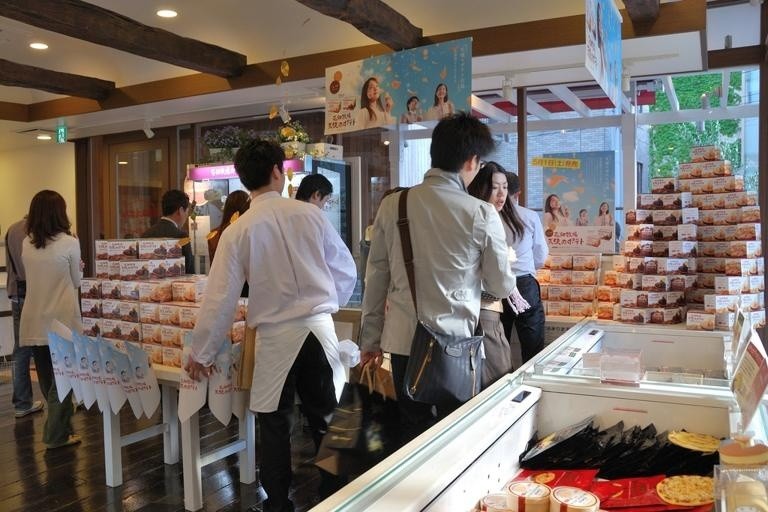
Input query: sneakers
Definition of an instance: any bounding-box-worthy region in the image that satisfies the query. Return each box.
[45,435,81,450]
[15,400,43,418]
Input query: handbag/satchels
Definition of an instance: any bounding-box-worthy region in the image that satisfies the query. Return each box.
[313,363,408,478]
[17,280,25,298]
[402,319,484,406]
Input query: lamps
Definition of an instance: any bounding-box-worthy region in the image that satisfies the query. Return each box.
[501,73,515,99]
[142,115,155,138]
[279,104,291,123]
[382,132,392,145]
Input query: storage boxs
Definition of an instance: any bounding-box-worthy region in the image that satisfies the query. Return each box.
[532,141,765,332]
[77,237,250,376]
[307,143,344,161]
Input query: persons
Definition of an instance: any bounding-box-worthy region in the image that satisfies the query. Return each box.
[542,195,614,247]
[141,190,197,275]
[195,189,223,230]
[358,112,548,443]
[18,190,86,449]
[206,190,249,297]
[352,77,455,130]
[6,213,44,418]
[184,138,357,512]
[295,174,332,210]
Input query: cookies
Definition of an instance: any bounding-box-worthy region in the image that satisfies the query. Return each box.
[329,81,341,94]
[334,70,344,83]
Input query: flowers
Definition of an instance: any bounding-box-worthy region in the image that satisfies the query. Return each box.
[200,120,309,163]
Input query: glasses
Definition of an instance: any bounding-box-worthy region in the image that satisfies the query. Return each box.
[479,159,487,170]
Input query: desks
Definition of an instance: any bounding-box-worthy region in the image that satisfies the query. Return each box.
[98,362,258,511]
